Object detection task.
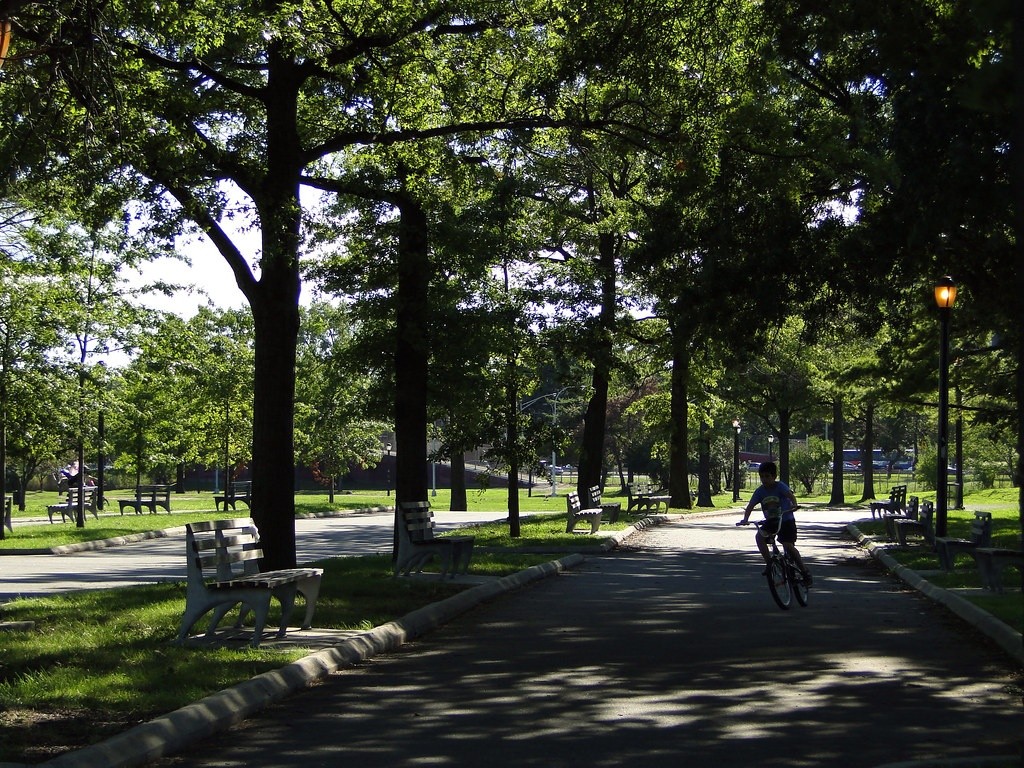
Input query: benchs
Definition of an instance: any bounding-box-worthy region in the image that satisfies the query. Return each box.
[639,483,672,514]
[894,500,934,546]
[974,547,1024,595]
[883,496,918,539]
[213,481,252,511]
[117,483,176,515]
[627,482,660,515]
[3,496,13,533]
[392,501,476,579]
[588,485,621,523]
[46,486,98,523]
[566,491,603,533]
[870,485,908,520]
[178,517,325,644]
[936,511,992,572]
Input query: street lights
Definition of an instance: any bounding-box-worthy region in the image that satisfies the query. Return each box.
[933,274,960,536]
[736,425,743,501]
[768,434,775,464]
[384,443,392,496]
[550,385,586,497]
[731,415,741,503]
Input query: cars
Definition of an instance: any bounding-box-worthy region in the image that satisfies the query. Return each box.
[747,462,762,472]
[947,464,957,476]
[549,466,564,477]
[829,459,863,474]
[891,461,911,471]
[871,460,889,473]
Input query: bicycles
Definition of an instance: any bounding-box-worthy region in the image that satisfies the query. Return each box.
[735,505,813,611]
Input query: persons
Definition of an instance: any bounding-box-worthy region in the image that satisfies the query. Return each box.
[739,462,815,578]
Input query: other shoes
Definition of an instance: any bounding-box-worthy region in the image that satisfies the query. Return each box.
[802,566,813,586]
[762,565,768,576]
